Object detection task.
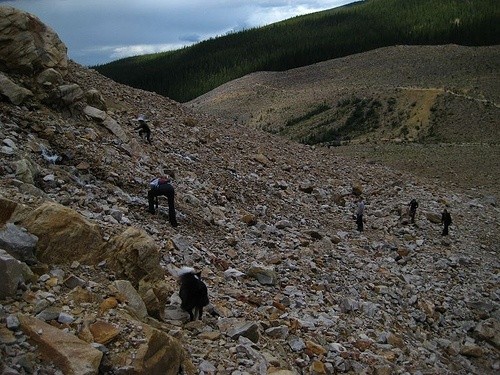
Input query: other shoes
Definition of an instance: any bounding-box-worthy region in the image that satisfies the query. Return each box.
[146,209,155,215]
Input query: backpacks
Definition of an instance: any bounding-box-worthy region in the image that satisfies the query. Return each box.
[157,176,170,185]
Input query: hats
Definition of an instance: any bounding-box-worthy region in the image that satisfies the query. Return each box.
[138,117,143,121]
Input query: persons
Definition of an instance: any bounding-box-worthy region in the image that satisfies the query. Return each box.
[134,118,152,145]
[409,199,418,224]
[148,176,178,227]
[356,197,364,231]
[441,209,452,236]
[180,275,209,320]
